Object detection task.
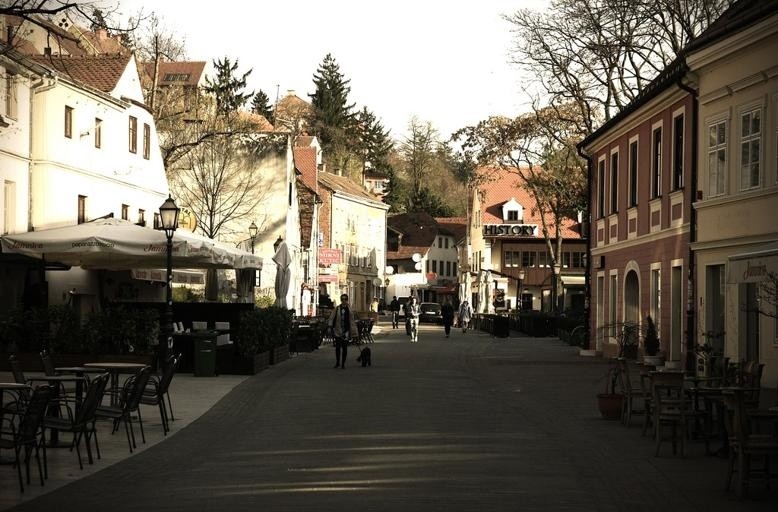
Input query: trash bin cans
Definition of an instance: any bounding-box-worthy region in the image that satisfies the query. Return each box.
[491,316,510,338]
[190,329,221,377]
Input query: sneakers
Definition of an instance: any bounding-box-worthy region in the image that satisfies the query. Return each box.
[410,338,414,342]
[414,336,418,343]
[341,364,347,370]
[333,362,341,368]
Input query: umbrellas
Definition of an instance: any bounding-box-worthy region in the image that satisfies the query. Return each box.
[272,241,293,310]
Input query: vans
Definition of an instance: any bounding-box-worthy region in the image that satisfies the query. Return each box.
[397,296,410,318]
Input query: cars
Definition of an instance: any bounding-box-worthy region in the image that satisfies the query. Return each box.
[419,302,444,326]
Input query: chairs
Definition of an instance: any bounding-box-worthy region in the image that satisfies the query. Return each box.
[0,345,183,494]
[613,355,777,504]
[297,314,376,347]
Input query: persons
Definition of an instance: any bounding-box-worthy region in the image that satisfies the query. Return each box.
[441,298,454,338]
[368,297,379,326]
[459,299,473,333]
[402,296,423,343]
[372,298,379,313]
[391,295,400,329]
[327,294,359,370]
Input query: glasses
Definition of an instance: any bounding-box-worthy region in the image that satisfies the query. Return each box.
[341,297,348,301]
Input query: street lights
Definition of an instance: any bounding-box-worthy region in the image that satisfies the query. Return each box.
[553,263,561,312]
[156,192,182,359]
[249,221,258,255]
[517,270,525,312]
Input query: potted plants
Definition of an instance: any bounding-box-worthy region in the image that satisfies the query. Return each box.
[0,300,161,375]
[266,304,297,365]
[595,318,653,421]
[215,308,270,376]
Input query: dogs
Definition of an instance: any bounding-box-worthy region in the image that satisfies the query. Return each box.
[356,347,371,367]
